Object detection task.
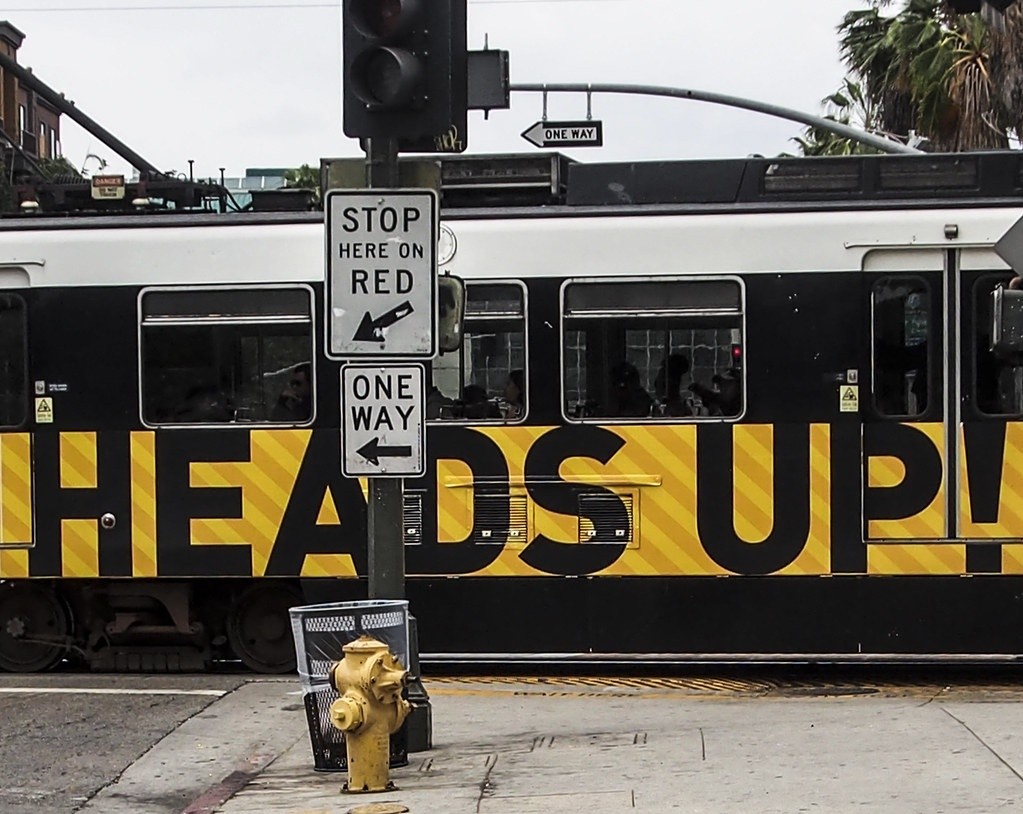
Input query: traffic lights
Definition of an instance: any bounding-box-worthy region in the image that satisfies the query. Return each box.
[341,1,453,137]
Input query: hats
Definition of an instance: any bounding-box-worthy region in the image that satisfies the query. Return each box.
[713,369,740,382]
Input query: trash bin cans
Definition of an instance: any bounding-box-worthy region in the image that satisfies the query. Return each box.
[287,599,411,771]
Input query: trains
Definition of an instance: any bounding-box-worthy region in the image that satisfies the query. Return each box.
[2,149,1023,669]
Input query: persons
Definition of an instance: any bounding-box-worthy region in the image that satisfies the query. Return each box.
[159,351,741,421]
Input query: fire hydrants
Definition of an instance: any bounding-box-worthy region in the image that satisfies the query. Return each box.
[327,633,421,795]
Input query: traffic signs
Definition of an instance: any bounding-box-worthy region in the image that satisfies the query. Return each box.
[325,186,440,362]
[338,362,427,479]
[521,121,604,148]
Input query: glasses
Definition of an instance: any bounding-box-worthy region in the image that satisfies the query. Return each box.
[288,380,303,388]
